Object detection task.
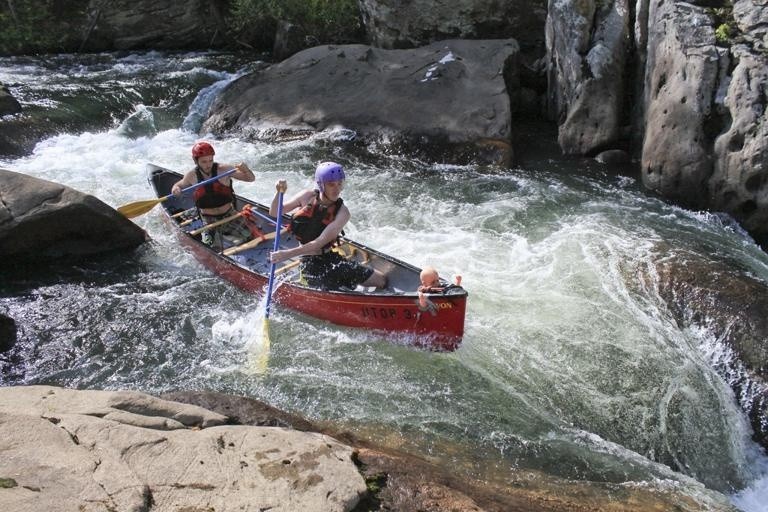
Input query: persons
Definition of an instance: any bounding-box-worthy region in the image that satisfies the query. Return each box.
[270,161,386,291]
[170,142,256,248]
[417,267,462,293]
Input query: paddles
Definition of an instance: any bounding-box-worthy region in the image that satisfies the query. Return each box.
[259,184,283,368]
[117,166,239,218]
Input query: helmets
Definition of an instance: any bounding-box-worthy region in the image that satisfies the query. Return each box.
[192,142,215,158]
[315,162,345,193]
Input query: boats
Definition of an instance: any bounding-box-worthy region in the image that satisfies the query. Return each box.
[146,163,468,352]
